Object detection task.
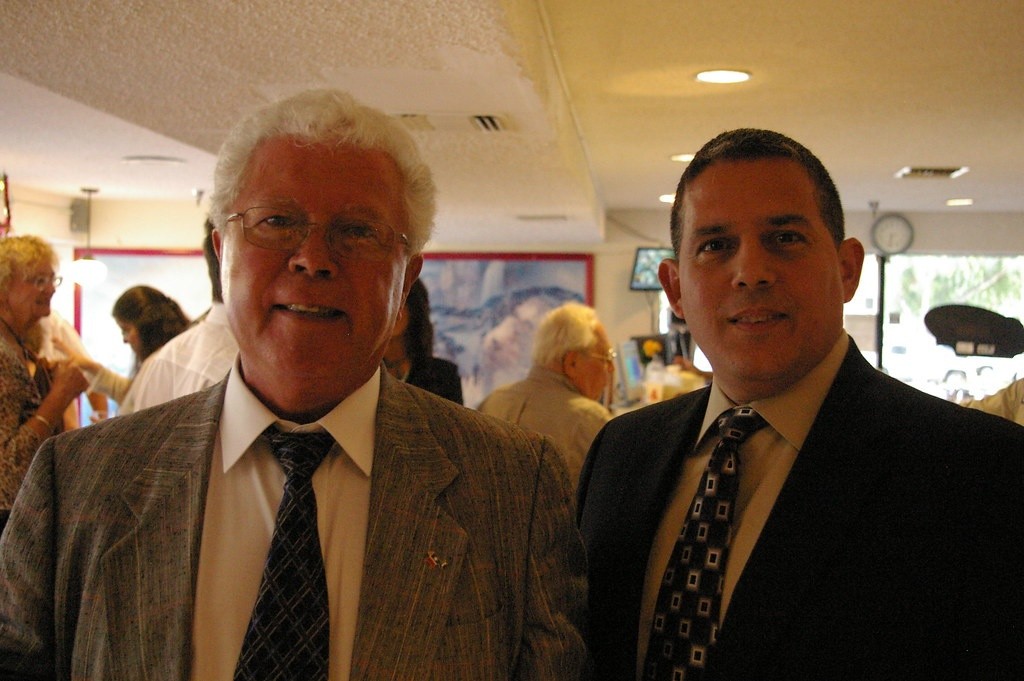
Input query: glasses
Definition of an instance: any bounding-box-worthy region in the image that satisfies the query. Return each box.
[222,206,411,262]
[576,348,616,370]
[12,276,62,292]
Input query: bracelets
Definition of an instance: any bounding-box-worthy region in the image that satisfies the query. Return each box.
[36,415,54,435]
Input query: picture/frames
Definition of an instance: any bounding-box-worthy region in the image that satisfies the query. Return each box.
[73,248,595,430]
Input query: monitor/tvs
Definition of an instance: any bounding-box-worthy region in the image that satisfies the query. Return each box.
[630,247,676,291]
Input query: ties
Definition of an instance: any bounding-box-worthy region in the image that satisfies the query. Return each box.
[639,407,770,681]
[231,425,336,681]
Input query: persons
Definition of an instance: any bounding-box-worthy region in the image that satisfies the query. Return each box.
[0,235,87,533]
[573,127,1024,681]
[35,312,109,435]
[119,216,240,423]
[51,285,190,403]
[473,301,615,492]
[382,279,463,407]
[0,88,591,681]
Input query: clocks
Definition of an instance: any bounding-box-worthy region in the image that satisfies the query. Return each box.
[870,214,914,255]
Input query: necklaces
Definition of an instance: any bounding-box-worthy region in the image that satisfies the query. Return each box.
[0,317,29,360]
[384,352,406,380]
